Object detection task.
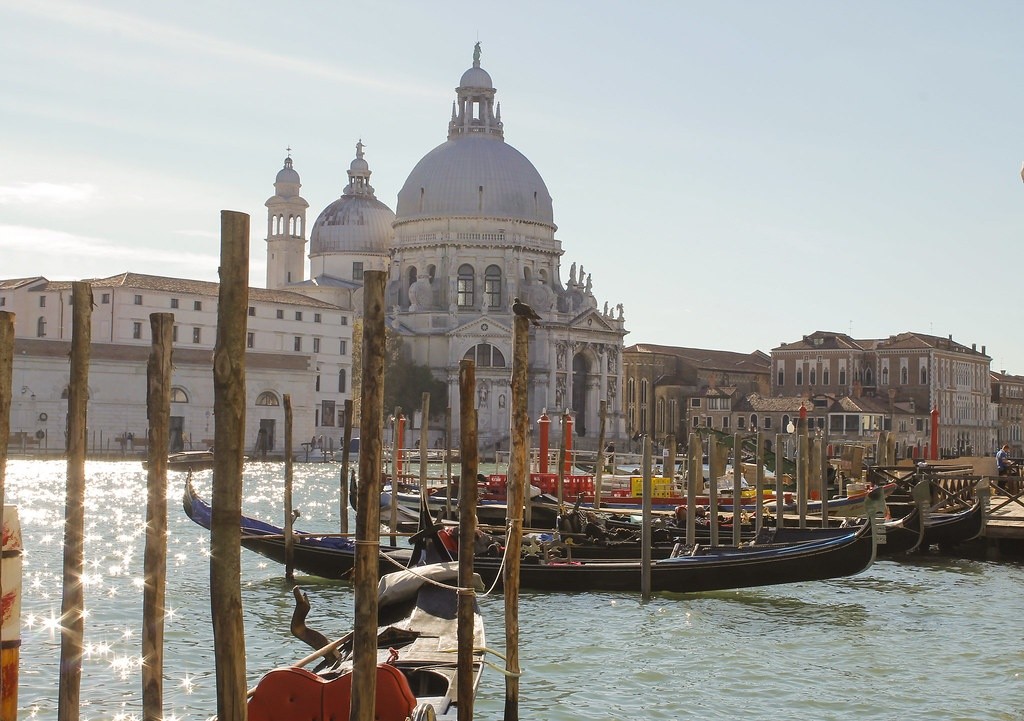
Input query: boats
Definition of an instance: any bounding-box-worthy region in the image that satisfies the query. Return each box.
[202,485,488,721]
[140,450,251,473]
[182,432,992,590]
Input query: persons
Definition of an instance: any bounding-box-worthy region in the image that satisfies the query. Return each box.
[996,444,1012,495]
[827,462,853,489]
[604,442,614,463]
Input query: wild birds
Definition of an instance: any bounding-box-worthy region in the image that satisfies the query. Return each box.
[513,297,542,326]
